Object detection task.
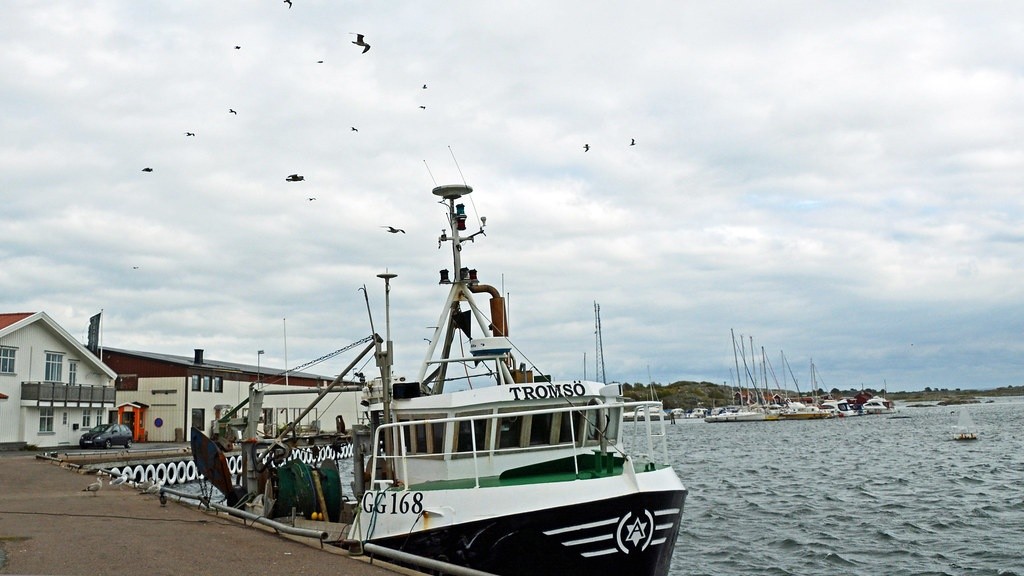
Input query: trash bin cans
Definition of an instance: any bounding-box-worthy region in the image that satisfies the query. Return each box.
[313,421,320,428]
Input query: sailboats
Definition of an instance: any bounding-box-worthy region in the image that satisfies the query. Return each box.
[704,328,867,424]
[623,361,670,421]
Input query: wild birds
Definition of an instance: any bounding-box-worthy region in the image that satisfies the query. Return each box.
[317,58,636,153]
[235,45,241,49]
[309,197,316,201]
[286,174,305,182]
[142,168,153,172]
[284,0,293,9]
[229,109,236,115]
[387,226,405,234]
[351,33,370,54]
[109,472,168,507]
[184,132,195,137]
[81,474,105,499]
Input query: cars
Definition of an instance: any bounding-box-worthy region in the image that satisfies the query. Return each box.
[79,423,133,449]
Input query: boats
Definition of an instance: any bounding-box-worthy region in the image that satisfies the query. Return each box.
[670,402,709,420]
[192,144,689,576]
[863,395,901,415]
